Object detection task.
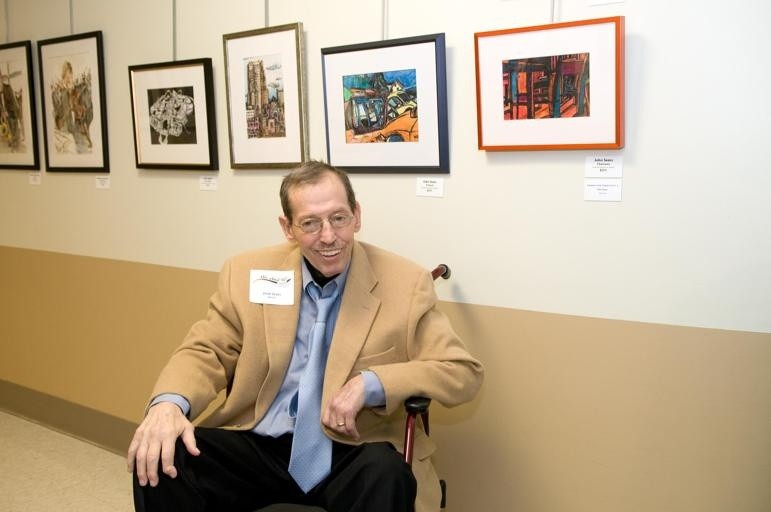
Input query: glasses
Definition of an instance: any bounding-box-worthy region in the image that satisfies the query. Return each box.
[292,213,353,234]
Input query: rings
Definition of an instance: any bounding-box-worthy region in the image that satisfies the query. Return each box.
[337,423,346,426]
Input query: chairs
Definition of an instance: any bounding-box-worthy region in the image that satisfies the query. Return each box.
[398,263,451,510]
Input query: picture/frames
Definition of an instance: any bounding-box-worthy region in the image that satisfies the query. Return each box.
[127,56,220,169]
[36,29,111,173]
[319,32,449,175]
[473,16,625,150]
[0,39,41,171]
[223,22,309,169]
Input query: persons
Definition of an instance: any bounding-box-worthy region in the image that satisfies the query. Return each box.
[127,160,483,511]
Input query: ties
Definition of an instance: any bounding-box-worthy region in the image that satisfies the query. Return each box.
[288,283,339,494]
